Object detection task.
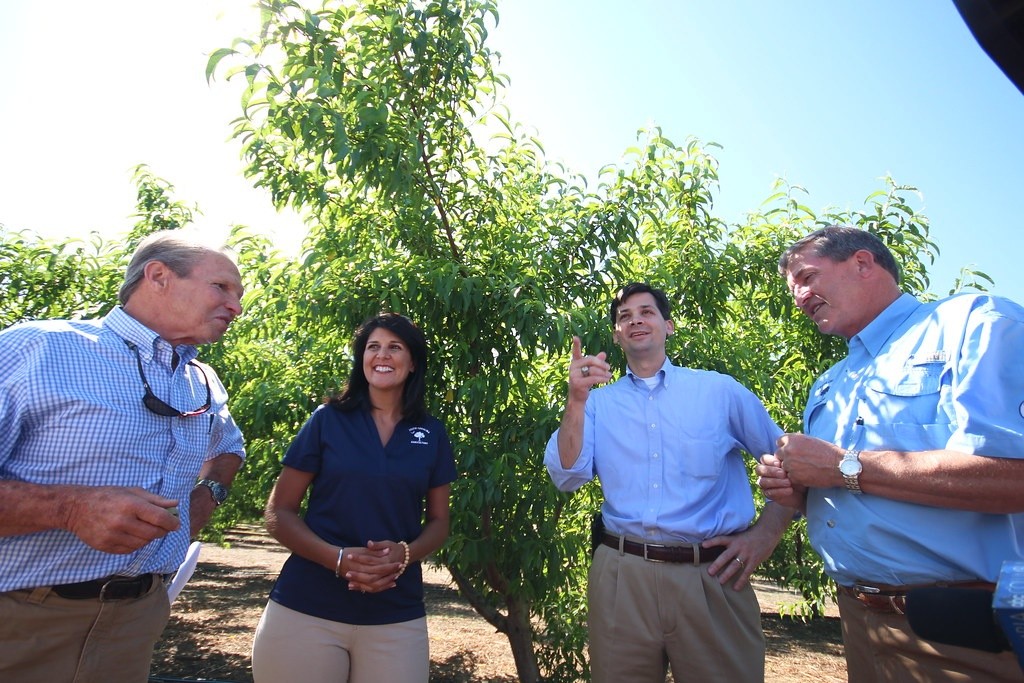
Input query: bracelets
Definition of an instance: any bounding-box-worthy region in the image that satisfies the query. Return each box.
[335,546,344,579]
[396,540,410,577]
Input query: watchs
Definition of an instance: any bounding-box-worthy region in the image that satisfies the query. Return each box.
[837,450,865,494]
[197,479,227,506]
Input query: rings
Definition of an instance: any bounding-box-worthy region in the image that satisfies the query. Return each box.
[734,556,743,568]
[582,366,590,375]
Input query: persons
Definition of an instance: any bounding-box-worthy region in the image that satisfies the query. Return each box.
[756,224,1024,682]
[543,283,806,683]
[251,313,458,683]
[0,230,247,682]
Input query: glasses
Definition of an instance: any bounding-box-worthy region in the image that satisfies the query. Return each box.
[124,339,211,418]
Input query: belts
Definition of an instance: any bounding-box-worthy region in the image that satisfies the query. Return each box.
[600,532,728,563]
[843,582,996,614]
[51,574,153,602]
